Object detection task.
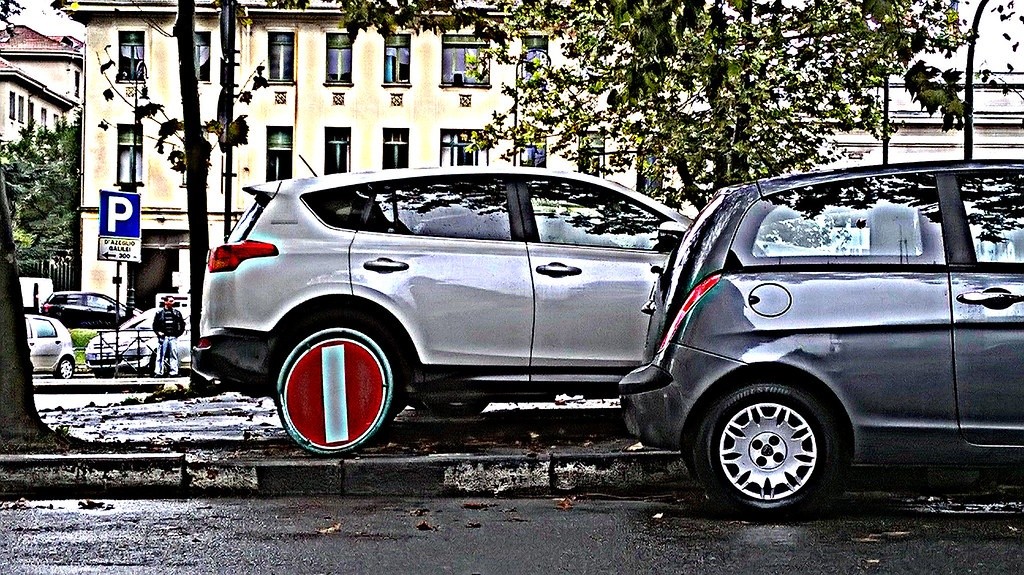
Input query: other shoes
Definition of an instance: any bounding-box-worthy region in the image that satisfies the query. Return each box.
[154,374,161,377]
[170,374,181,378]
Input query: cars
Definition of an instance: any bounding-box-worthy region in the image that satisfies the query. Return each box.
[84,306,190,378]
[24,314,75,380]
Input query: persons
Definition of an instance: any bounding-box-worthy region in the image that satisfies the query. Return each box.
[153,295,187,378]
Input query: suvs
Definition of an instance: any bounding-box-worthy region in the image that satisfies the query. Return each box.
[42,290,144,329]
[619,160,1023,516]
[190,166,839,458]
